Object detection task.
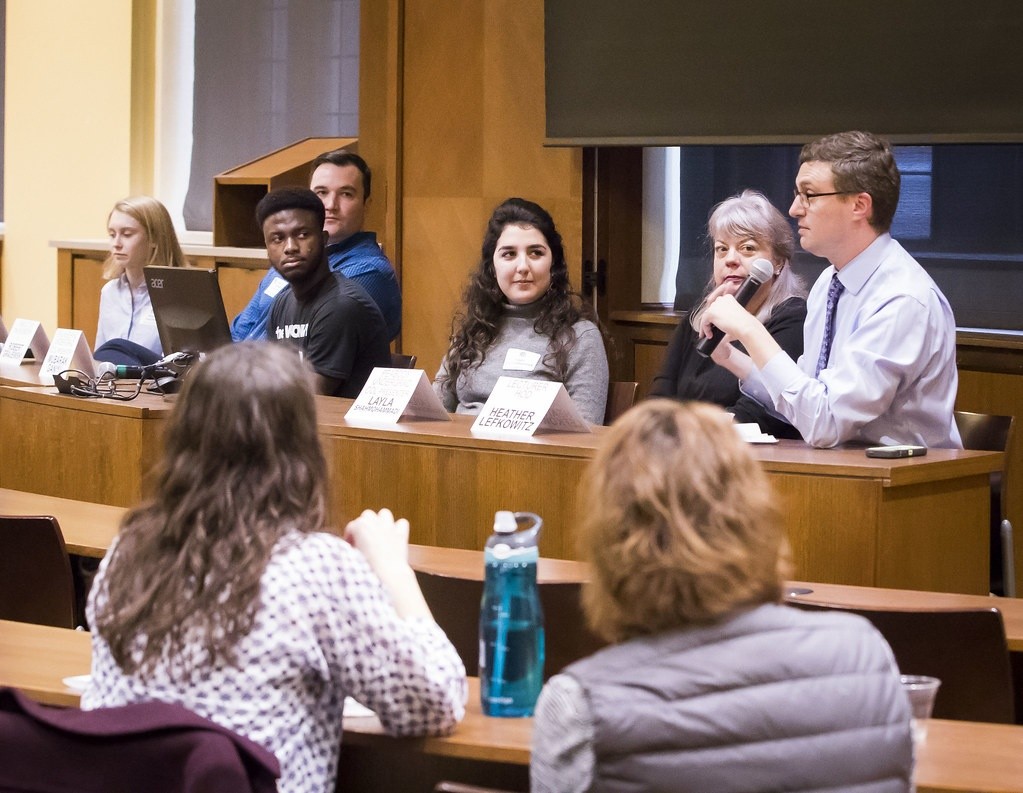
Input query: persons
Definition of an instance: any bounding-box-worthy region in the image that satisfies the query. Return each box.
[84,335,468,793]
[647,187,811,439]
[431,198,609,426]
[230,148,403,345]
[255,184,391,399]
[698,133,965,451]
[529,396,914,792]
[94,194,193,366]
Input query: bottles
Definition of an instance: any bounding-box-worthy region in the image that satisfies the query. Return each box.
[480,510,545,718]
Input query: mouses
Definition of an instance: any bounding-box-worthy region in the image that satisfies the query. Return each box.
[147,376,182,393]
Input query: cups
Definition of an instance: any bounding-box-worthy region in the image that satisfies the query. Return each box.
[901,675,941,745]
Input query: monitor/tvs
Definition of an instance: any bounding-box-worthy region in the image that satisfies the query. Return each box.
[143,265,233,375]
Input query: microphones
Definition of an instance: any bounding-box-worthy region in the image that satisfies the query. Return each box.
[97,361,169,380]
[696,259,773,357]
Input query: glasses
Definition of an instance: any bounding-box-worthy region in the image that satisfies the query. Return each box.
[793,186,874,209]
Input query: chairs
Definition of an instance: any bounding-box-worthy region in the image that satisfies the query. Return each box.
[951,411,1015,597]
[784,595,1014,724]
[403,568,606,691]
[1,517,76,628]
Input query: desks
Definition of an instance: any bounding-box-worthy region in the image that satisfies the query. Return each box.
[1,481,1023,793]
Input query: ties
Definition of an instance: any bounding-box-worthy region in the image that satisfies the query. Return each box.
[815,274,845,380]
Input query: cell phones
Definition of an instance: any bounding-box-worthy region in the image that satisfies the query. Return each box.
[865,445,927,458]
